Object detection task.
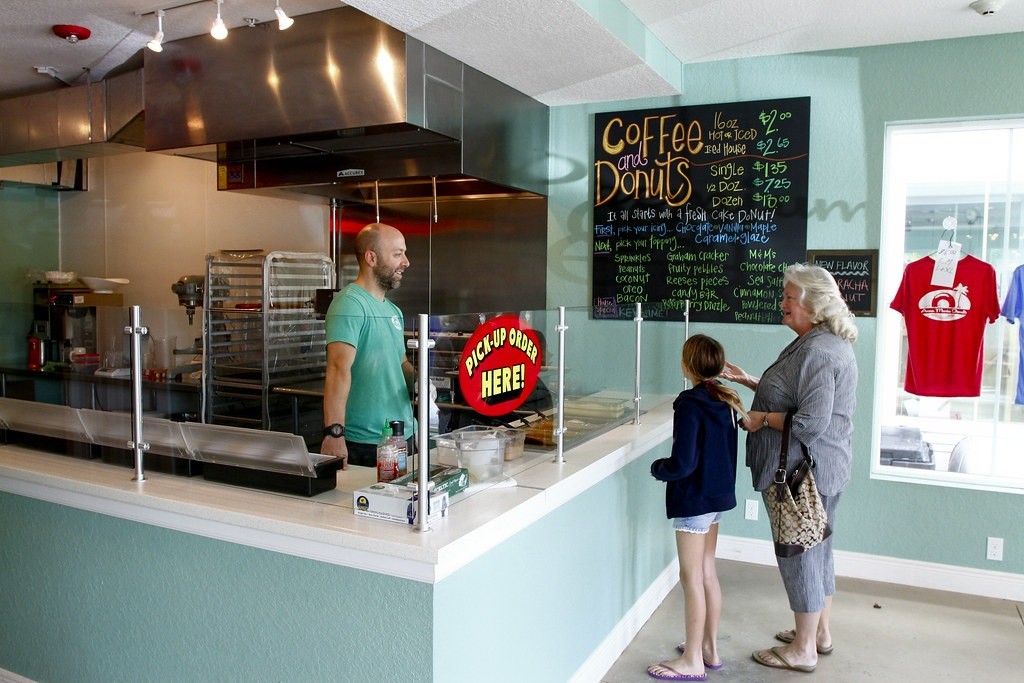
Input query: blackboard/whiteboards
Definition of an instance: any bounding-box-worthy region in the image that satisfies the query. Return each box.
[593,96,811,325]
[807,249,879,317]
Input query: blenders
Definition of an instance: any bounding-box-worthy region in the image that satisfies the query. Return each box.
[170,274,230,354]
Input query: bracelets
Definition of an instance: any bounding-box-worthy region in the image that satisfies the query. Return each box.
[762,415,769,427]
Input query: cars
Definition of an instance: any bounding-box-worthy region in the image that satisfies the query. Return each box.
[881,423,936,470]
[904,389,1024,422]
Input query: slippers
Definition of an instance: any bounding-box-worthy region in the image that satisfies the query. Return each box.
[678,641,723,670]
[647,660,708,681]
[776,629,833,654]
[752,647,816,673]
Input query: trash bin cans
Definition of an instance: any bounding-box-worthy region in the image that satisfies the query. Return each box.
[879,425,935,469]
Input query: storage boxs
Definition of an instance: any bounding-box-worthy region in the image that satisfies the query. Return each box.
[0,394,528,526]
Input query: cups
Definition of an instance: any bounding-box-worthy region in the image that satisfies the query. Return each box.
[69,347,100,364]
[154,335,176,368]
[103,351,122,368]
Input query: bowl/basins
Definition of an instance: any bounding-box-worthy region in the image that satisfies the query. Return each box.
[46,271,75,285]
[78,277,130,294]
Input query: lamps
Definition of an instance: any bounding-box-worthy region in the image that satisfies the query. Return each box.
[146,0,294,53]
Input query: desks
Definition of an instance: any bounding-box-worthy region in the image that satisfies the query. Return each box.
[3,362,537,462]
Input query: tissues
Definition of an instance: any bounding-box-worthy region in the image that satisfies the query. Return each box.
[353,481,449,526]
[386,462,469,498]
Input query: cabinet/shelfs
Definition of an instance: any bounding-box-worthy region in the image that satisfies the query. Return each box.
[205,249,338,430]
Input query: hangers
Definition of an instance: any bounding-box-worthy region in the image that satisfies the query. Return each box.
[928,228,966,258]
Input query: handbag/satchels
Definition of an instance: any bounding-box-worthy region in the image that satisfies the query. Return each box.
[762,410,833,557]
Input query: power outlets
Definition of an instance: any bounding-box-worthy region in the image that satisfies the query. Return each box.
[987,536,1004,561]
[744,499,759,520]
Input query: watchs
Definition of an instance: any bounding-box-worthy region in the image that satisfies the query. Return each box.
[322,423,346,438]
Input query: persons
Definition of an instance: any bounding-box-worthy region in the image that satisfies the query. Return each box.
[321,223,437,471]
[717,261,858,673]
[646,333,738,681]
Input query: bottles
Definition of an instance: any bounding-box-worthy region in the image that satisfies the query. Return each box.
[390,421,408,476]
[26,330,69,371]
[143,366,173,383]
[377,419,398,484]
